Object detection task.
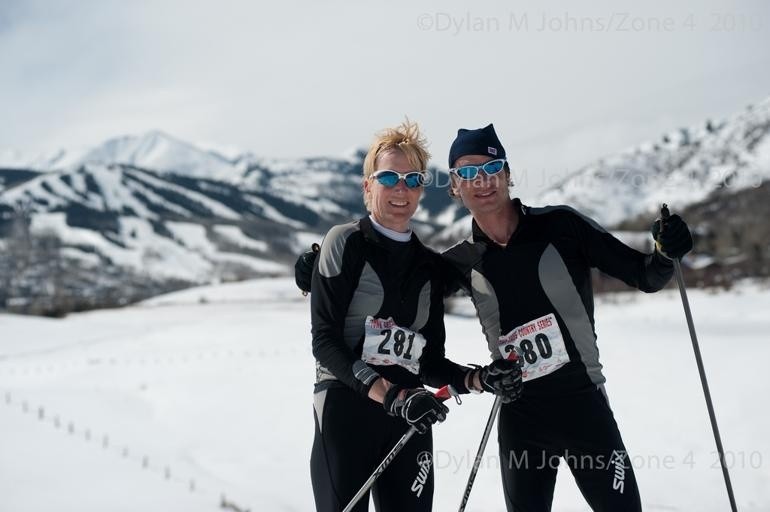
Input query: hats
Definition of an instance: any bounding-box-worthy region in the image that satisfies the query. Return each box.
[449,123,508,168]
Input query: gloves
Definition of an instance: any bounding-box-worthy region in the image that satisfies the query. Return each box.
[383,384,448,435]
[295,253,318,292]
[479,358,525,404]
[652,213,693,265]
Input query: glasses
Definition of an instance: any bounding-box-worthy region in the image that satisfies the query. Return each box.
[449,157,508,182]
[368,169,426,190]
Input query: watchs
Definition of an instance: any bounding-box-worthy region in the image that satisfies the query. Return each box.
[466,362,485,395]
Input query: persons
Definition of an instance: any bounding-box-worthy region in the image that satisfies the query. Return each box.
[308,113,526,512]
[291,123,696,512]
